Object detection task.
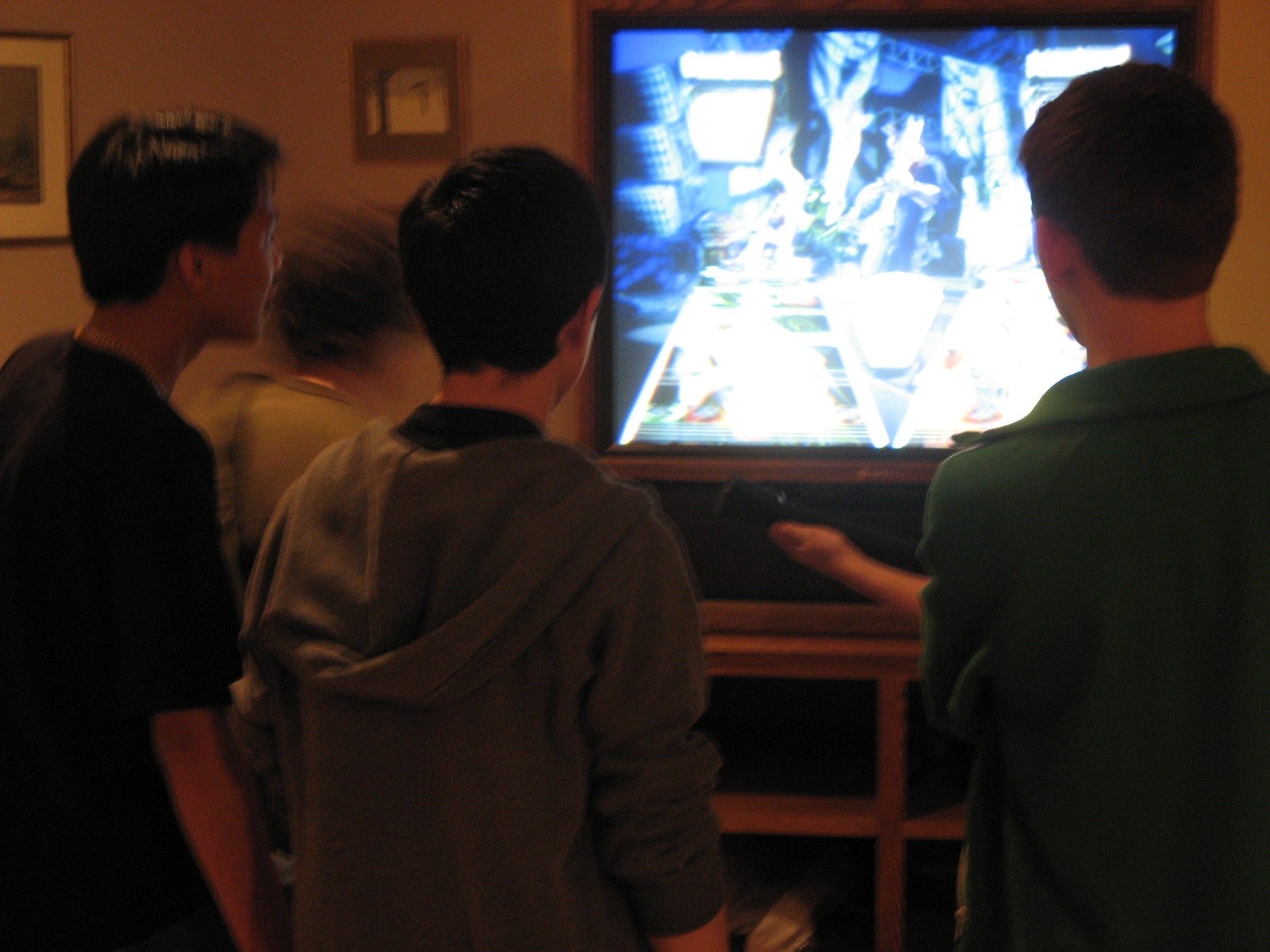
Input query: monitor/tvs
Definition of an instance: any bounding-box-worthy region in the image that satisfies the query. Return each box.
[589,5,1198,461]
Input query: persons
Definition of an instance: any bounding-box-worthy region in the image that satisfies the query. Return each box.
[917,63,1270,952]
[2,108,283,949]
[767,520,932,606]
[170,202,422,613]
[237,142,731,951]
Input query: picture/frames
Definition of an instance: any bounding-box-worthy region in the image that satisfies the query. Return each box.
[346,33,469,167]
[0,28,77,244]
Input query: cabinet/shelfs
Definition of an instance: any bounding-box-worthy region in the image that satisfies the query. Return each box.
[707,642,971,951]
